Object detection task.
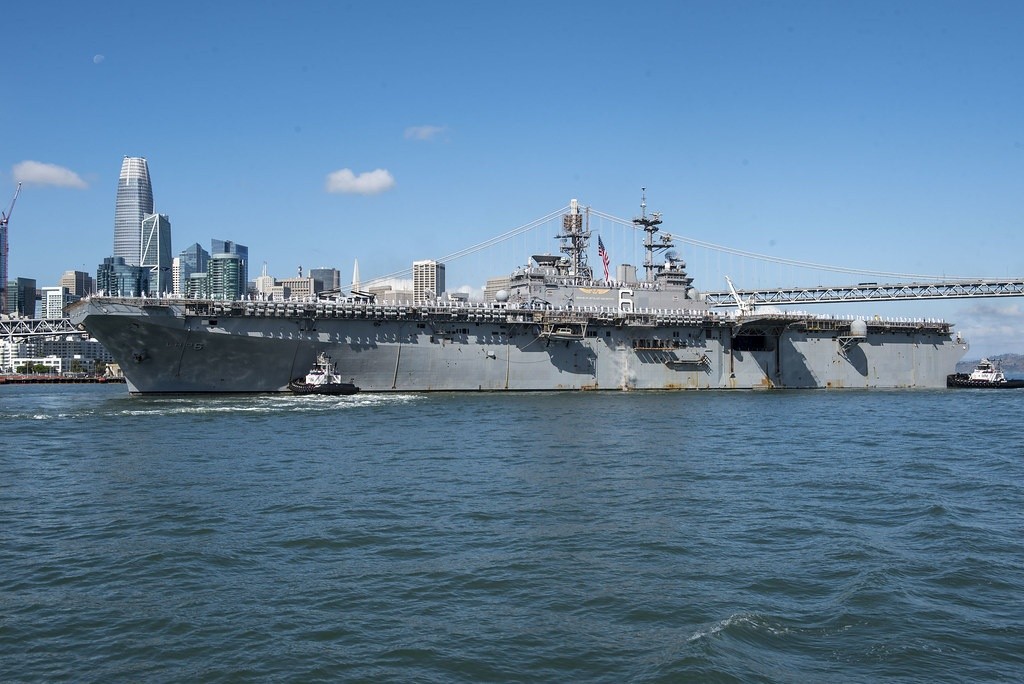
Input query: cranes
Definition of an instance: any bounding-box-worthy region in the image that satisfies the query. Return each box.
[2,181,25,226]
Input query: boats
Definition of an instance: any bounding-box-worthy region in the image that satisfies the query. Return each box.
[287,349,362,396]
[946,355,1024,389]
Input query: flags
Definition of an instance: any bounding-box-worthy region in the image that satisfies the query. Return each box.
[598,235,611,281]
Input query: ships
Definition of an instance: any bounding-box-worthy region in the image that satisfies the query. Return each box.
[58,194,1024,392]
